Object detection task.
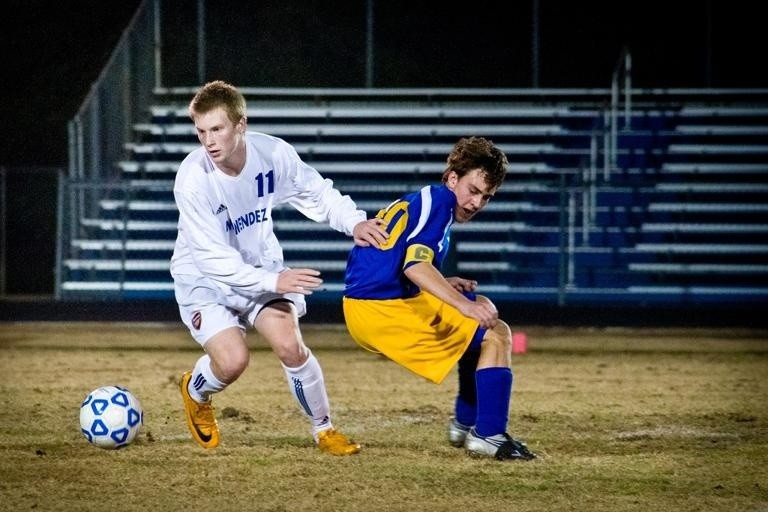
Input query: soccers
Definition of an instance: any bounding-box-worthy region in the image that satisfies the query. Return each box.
[80,387,143,449]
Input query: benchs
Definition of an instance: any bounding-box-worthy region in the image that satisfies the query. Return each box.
[62,86,768,303]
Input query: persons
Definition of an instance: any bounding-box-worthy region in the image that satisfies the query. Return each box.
[342,136,538,462]
[170,79,391,457]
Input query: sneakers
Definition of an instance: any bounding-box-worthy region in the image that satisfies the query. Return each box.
[448,417,536,461]
[315,427,362,457]
[180,371,221,449]
